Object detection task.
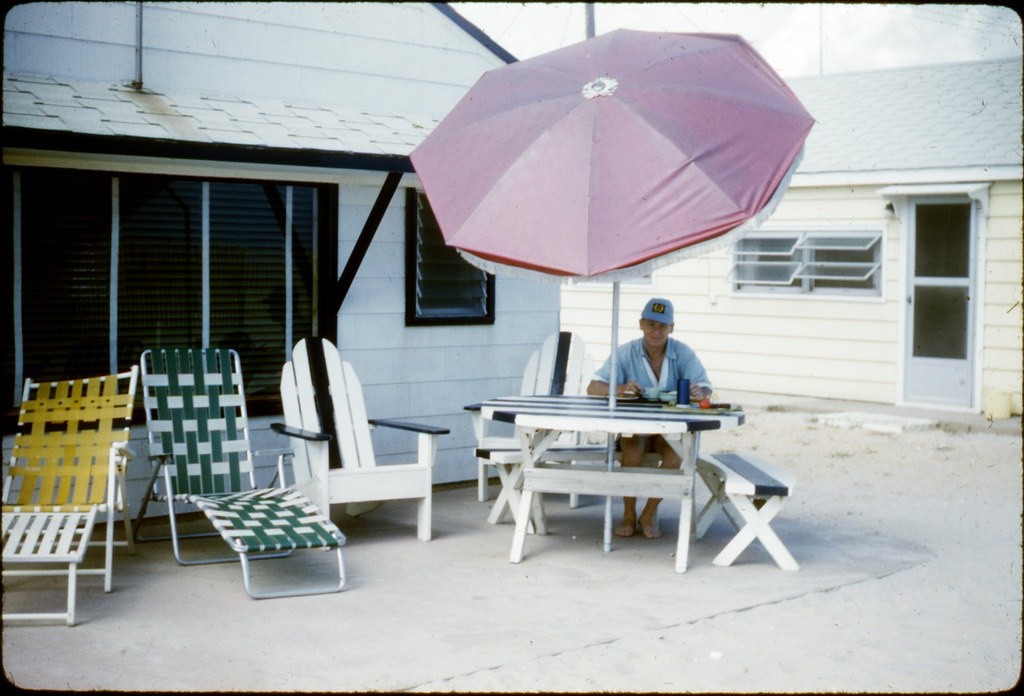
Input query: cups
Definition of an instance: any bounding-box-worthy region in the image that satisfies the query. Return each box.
[677,379,690,409]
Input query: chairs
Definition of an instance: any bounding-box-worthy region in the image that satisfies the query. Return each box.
[131,347,348,600]
[464,331,599,509]
[269,338,451,540]
[1,362,139,628]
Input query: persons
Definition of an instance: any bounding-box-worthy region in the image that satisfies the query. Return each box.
[588,297,713,537]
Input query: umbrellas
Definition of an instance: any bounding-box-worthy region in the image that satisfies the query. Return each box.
[412,32,815,552]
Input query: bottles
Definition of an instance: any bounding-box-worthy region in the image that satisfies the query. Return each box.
[700,397,709,408]
[713,393,719,408]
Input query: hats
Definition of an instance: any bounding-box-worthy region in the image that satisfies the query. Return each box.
[642,298,674,324]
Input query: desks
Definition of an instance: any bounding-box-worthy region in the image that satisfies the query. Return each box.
[480,395,746,573]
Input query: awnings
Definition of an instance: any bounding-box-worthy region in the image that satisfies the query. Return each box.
[879,182,991,217]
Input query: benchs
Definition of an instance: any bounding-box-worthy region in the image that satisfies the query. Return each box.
[698,452,802,573]
[473,446,614,535]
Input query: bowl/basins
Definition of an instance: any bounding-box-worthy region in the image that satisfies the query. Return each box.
[645,387,667,396]
[659,392,678,401]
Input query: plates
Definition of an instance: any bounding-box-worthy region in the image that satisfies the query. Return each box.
[643,396,661,401]
[605,396,639,400]
[690,396,702,407]
